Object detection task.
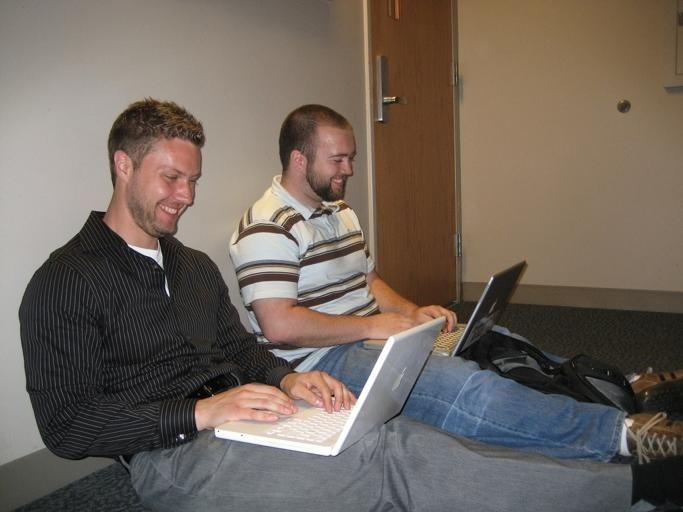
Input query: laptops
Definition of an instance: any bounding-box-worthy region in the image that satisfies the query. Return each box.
[215,317,447,457]
[362,261,528,358]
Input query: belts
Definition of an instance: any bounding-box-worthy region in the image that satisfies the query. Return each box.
[118,369,250,472]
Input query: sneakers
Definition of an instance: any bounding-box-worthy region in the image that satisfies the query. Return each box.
[628,369,683,414]
[616,413,683,465]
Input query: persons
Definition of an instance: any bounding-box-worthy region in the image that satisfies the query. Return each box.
[15,96,681,511]
[227,103,682,465]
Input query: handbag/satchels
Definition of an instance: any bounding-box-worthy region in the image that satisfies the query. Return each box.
[465,330,637,412]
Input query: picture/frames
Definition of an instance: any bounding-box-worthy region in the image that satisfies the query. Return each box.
[664,0,683,88]
